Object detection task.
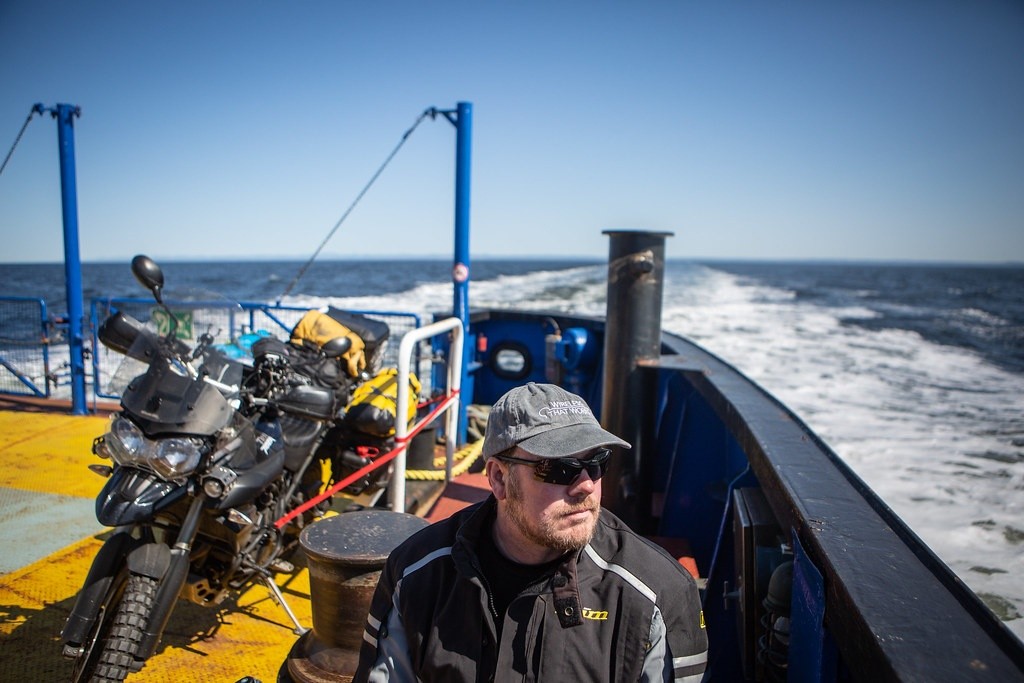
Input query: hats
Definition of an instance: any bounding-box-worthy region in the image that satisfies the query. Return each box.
[482,382,632,462]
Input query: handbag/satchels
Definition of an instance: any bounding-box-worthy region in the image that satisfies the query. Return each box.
[291,307,366,382]
[344,364,422,448]
[213,327,276,364]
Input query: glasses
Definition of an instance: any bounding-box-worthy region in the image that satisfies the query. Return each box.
[494,449,612,485]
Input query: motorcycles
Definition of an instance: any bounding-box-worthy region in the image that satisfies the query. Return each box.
[60,253,421,683]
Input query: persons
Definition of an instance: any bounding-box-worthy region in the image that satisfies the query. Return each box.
[352,380,709,683]
